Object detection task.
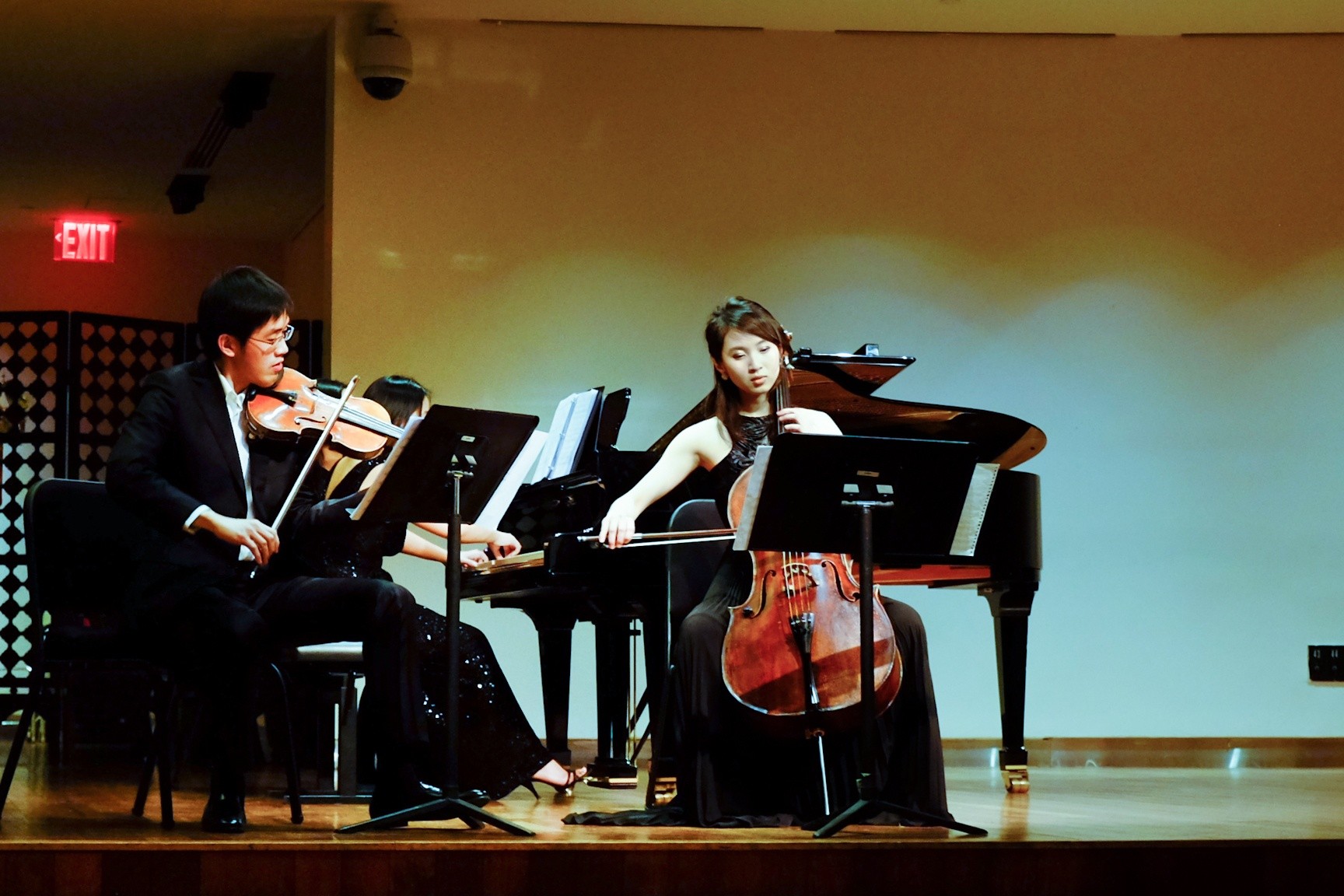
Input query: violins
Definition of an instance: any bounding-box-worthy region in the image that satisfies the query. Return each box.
[243,367,405,461]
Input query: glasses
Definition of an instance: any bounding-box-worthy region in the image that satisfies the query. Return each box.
[245,324,294,346]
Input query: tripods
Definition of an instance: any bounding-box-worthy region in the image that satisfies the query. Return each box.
[749,432,990,837]
[335,406,539,837]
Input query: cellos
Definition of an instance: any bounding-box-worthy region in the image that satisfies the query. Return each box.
[717,325,907,817]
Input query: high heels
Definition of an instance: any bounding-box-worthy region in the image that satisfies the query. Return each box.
[517,758,589,798]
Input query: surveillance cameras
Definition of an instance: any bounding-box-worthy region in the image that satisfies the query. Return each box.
[357,35,414,100]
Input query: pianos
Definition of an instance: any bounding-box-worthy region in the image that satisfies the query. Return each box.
[456,346,1047,793]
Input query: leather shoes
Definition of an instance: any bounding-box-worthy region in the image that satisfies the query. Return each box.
[368,779,490,826]
[199,784,248,834]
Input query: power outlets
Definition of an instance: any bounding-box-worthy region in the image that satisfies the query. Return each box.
[1307,644,1344,683]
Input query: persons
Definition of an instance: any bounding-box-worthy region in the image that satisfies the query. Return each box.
[563,297,955,827]
[326,375,587,821]
[101,267,488,832]
[303,374,344,575]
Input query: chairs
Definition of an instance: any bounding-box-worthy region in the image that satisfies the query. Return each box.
[643,497,727,817]
[0,472,304,833]
